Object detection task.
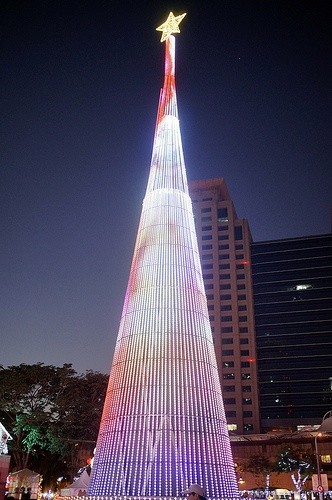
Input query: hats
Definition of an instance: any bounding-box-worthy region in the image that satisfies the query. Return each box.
[180,484,208,499]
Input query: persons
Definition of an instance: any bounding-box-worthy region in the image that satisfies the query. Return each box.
[240,490,266,500]
[184,485,210,500]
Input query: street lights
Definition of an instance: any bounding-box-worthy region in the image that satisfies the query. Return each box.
[314,432,324,491]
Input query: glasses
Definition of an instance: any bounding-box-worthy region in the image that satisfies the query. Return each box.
[183,492,196,497]
